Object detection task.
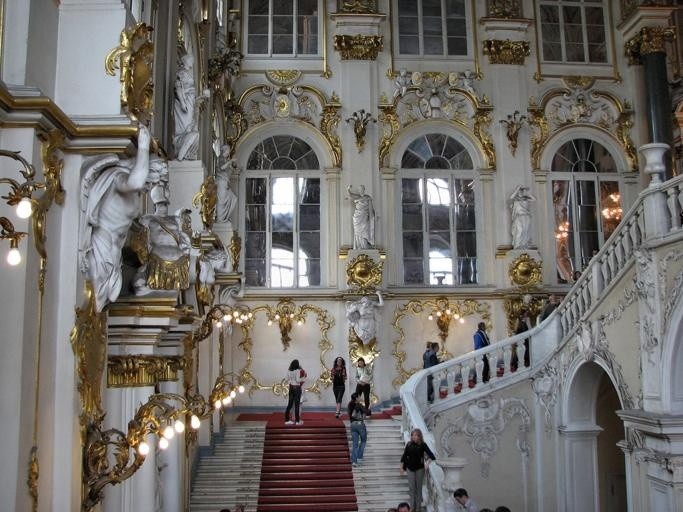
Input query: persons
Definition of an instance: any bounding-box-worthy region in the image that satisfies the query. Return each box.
[281,355,376,426]
[214,503,247,511]
[398,428,435,510]
[421,295,566,404]
[569,269,585,282]
[346,392,373,468]
[83,51,241,309]
[588,248,601,259]
[508,186,538,251]
[344,182,385,356]
[380,486,513,512]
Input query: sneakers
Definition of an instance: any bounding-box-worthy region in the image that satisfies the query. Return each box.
[284,421,303,425]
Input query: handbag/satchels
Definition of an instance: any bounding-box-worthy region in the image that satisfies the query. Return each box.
[351,410,364,420]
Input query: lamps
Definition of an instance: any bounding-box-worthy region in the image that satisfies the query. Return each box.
[267,310,305,349]
[120,391,203,454]
[426,304,463,345]
[210,304,253,329]
[207,372,248,409]
[0,149,39,220]
[1,216,29,265]
